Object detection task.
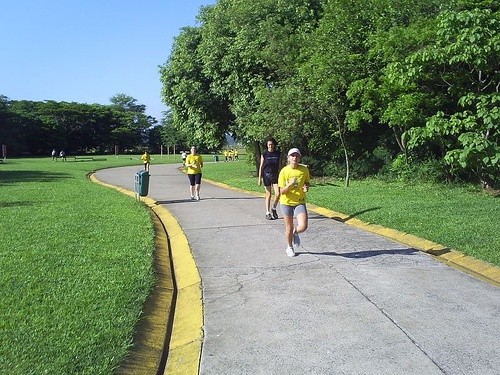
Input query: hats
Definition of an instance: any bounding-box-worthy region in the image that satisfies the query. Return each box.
[288,148,301,157]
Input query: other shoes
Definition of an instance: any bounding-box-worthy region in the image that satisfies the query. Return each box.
[196,195,200,200]
[149,174,151,176]
[191,195,195,200]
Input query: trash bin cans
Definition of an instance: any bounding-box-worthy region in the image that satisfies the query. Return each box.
[134,170,149,197]
[215,156,218,163]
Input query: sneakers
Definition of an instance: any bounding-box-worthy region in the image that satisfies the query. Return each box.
[271,208,278,219]
[286,247,295,257]
[293,231,300,247]
[266,213,273,220]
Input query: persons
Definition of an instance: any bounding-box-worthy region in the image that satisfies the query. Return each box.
[258,137,282,220]
[141,151,151,176]
[223,149,240,163]
[52,149,66,162]
[277,148,311,257]
[181,145,203,200]
